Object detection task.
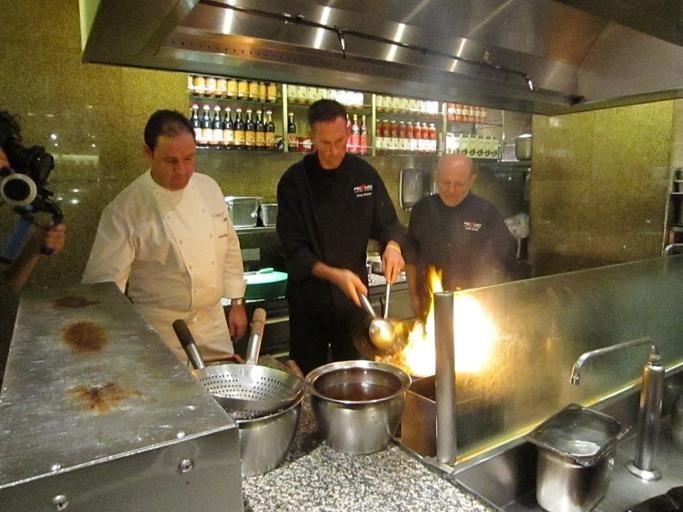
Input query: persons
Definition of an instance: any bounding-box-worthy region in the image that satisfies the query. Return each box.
[274,98,405,378]
[0,148,66,313]
[404,152,518,324]
[81,108,248,369]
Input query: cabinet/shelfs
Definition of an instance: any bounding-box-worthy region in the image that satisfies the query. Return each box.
[188,74,288,154]
[364,90,447,160]
[438,99,505,163]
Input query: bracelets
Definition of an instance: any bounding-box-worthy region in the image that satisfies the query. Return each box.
[387,244,402,253]
[230,298,245,305]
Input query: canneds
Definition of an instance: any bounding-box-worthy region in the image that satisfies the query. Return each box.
[416,99,424,117]
[475,106,481,121]
[308,87,317,106]
[425,101,433,120]
[469,106,475,122]
[217,79,228,97]
[482,106,488,124]
[288,85,297,104]
[462,104,469,120]
[456,104,463,121]
[297,86,307,105]
[401,98,408,113]
[194,77,206,94]
[260,82,268,102]
[238,79,249,101]
[338,90,347,103]
[448,103,455,121]
[376,94,383,111]
[392,97,401,113]
[347,92,355,105]
[206,77,216,96]
[228,79,238,99]
[186,75,193,93]
[355,93,364,105]
[318,88,327,102]
[408,99,417,115]
[328,89,337,101]
[250,80,260,101]
[383,95,392,114]
[431,101,438,119]
[268,82,279,103]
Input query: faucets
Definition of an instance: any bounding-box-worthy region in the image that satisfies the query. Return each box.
[569,337,662,482]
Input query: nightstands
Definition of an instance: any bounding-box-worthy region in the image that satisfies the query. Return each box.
[276,83,376,157]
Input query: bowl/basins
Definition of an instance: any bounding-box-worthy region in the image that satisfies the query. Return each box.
[302,359,413,456]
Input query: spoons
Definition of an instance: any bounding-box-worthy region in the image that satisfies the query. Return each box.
[368,284,398,348]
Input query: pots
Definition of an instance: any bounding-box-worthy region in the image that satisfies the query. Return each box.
[172,318,306,478]
[515,134,533,160]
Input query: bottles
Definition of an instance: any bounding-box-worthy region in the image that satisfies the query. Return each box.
[201,104,212,145]
[485,134,491,159]
[245,107,256,150]
[454,133,462,155]
[345,114,351,146]
[461,133,469,155]
[446,132,452,154]
[224,105,234,150]
[407,121,414,150]
[477,134,484,158]
[376,118,383,151]
[421,121,429,151]
[391,119,399,150]
[255,109,266,149]
[468,133,480,156]
[383,120,390,149]
[288,112,298,150]
[429,123,437,152]
[188,102,202,145]
[266,110,276,150]
[352,115,360,146]
[235,108,246,149]
[414,121,422,152]
[212,105,223,146]
[361,114,368,146]
[492,135,498,159]
[400,120,406,151]
